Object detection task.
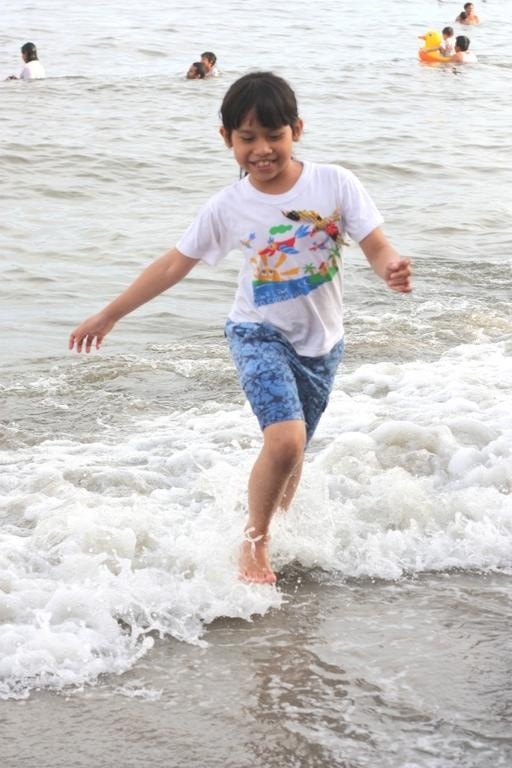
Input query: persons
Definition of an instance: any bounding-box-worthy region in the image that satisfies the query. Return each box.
[186,51,217,82]
[418,2,479,63]
[69,72,413,584]
[13,43,48,81]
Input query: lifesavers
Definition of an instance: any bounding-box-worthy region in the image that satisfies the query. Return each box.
[418,32,453,62]
